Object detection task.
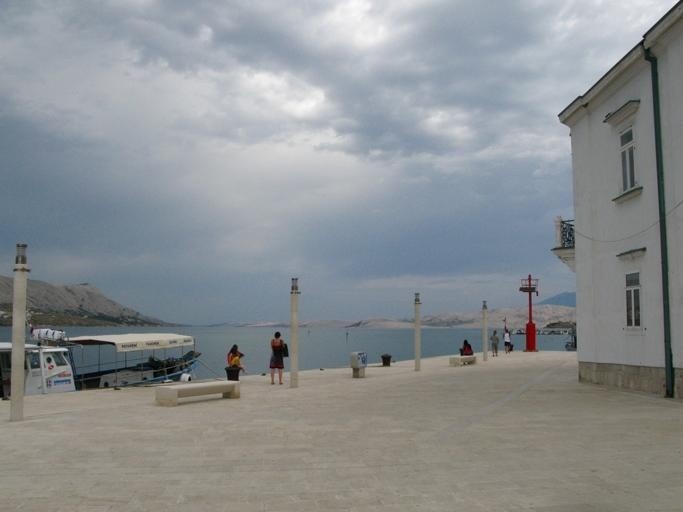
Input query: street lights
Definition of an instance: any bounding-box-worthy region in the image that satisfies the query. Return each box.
[481,298,491,361]
[8,239,30,423]
[288,275,302,391]
[412,291,424,373]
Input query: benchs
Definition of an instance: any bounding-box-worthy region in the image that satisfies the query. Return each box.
[448,355,476,369]
[155,380,242,407]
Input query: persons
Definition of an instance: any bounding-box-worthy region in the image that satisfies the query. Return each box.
[459,339,472,355]
[227,347,245,374]
[269,332,285,386]
[227,343,245,358]
[503,328,511,353]
[489,330,498,357]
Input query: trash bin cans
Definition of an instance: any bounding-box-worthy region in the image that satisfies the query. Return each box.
[381,354,392,367]
[510,344,513,351]
[225,367,240,381]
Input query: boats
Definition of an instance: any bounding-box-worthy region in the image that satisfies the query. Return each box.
[516,327,570,337]
[33,323,205,395]
[1,336,78,403]
[565,333,578,352]
